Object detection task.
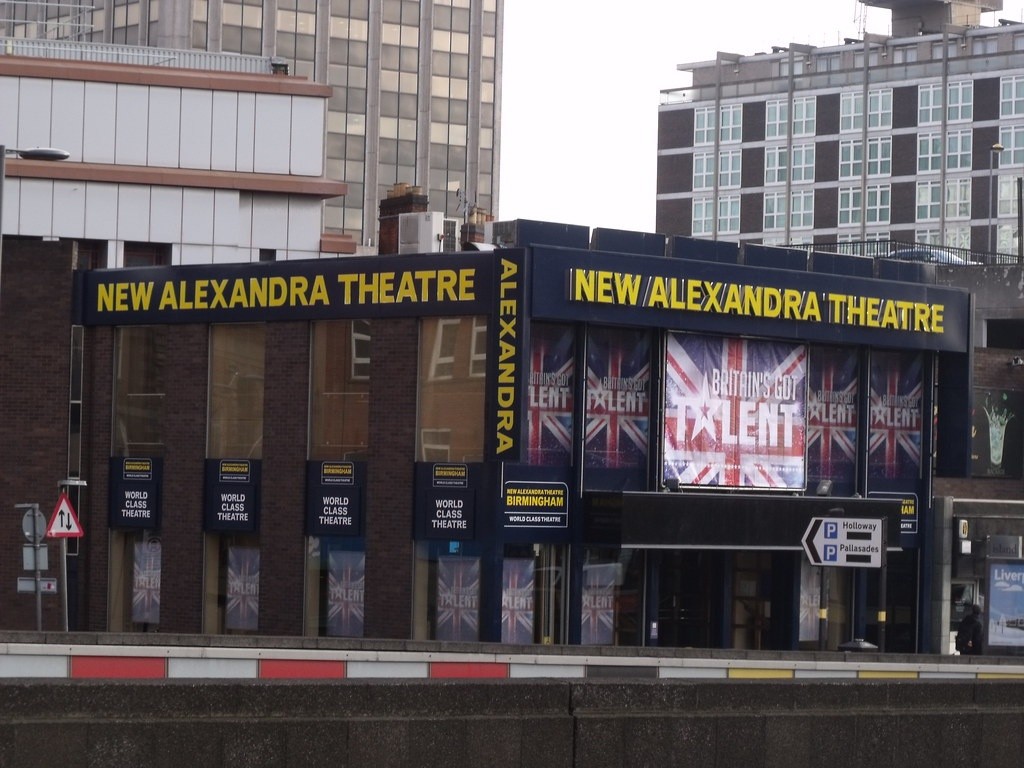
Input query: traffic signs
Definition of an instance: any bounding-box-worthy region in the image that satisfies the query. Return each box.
[800,515,882,570]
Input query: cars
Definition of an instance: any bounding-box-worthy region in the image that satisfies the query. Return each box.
[890,248,983,266]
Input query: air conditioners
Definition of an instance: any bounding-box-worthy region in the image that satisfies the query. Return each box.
[397,211,446,255]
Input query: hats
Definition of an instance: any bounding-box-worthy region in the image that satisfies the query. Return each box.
[973,605,981,615]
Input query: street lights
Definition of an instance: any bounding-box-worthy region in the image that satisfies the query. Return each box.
[988,142,1004,266]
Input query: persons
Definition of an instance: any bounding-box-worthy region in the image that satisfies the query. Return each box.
[956,605,983,655]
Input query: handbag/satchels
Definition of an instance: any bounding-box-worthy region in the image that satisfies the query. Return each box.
[956,636,973,652]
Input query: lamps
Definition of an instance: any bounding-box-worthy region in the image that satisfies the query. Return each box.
[663,478,680,494]
[816,479,833,498]
